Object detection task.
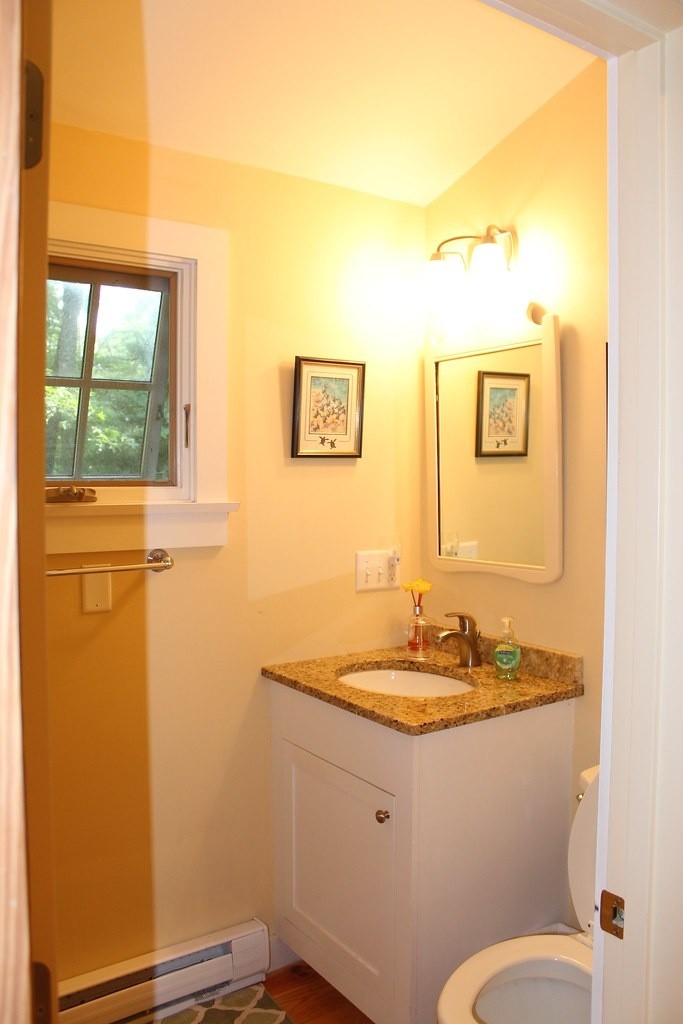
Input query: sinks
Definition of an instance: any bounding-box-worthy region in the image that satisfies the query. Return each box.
[334,659,479,698]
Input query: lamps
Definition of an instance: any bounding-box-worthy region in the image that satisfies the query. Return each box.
[430,225,516,284]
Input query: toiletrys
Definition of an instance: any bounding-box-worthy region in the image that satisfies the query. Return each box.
[493,617,522,681]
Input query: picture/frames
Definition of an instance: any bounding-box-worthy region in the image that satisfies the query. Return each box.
[475,371,531,456]
[290,356,365,458]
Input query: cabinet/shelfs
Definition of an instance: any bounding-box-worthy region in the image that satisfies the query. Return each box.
[272,681,574,1024]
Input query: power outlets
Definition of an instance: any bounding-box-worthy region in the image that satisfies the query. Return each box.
[383,550,401,589]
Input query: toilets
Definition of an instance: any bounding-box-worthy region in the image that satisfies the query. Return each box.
[435,767,601,1024]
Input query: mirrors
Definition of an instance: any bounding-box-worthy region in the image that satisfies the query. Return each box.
[423,314,563,585]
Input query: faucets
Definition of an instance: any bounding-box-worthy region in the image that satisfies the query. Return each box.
[436,612,483,668]
